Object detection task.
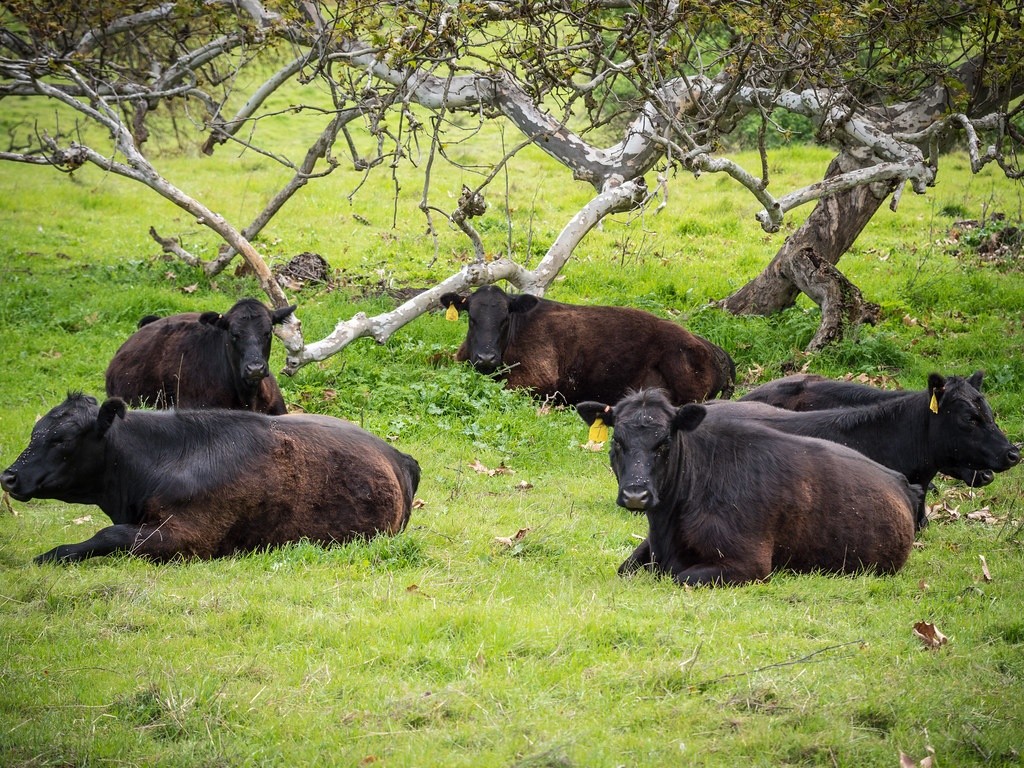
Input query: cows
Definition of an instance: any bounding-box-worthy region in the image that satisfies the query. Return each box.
[440,282,1024,590]
[0,294,423,571]
